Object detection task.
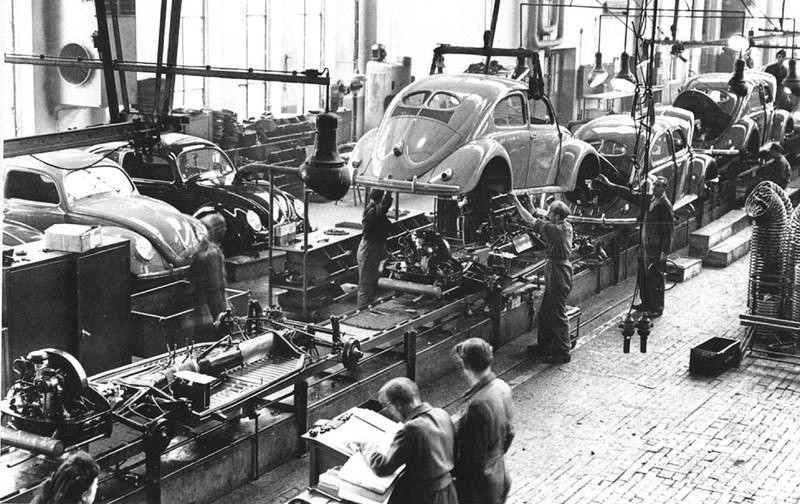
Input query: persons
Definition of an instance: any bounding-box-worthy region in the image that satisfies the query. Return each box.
[745,141,792,202]
[504,190,576,362]
[596,173,675,318]
[353,374,461,503]
[187,212,235,340]
[764,47,790,107]
[450,336,516,503]
[356,187,394,309]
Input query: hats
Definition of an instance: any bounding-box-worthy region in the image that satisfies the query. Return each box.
[453,337,494,364]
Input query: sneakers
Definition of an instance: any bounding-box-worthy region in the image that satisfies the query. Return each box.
[527,342,571,363]
[632,302,662,319]
